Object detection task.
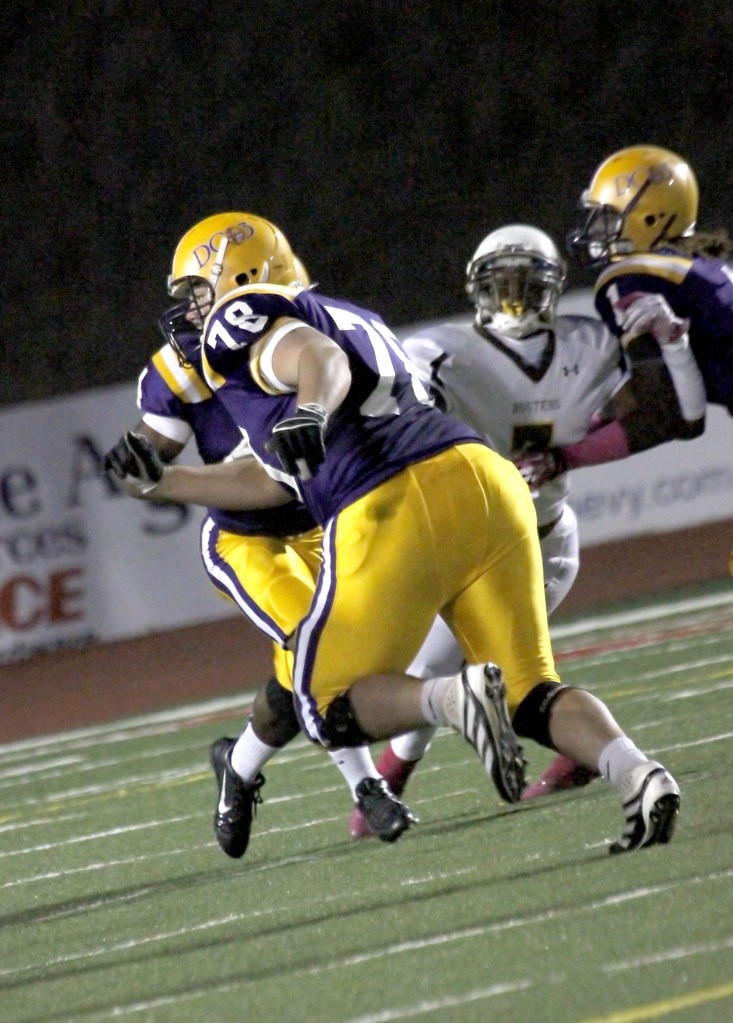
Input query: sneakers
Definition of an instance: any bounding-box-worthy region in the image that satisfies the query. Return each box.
[457,658,526,804]
[519,753,601,799]
[608,760,680,853]
[210,737,268,858]
[349,782,401,837]
[351,776,413,842]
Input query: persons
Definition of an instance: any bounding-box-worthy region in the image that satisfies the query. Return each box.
[511,143,733,490]
[102,332,417,859]
[350,222,707,838]
[106,208,680,857]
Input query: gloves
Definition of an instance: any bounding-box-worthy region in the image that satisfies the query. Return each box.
[511,442,557,494]
[101,430,163,497]
[263,402,326,481]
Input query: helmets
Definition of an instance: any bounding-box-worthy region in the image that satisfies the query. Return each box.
[157,211,314,366]
[568,145,700,272]
[466,223,566,336]
